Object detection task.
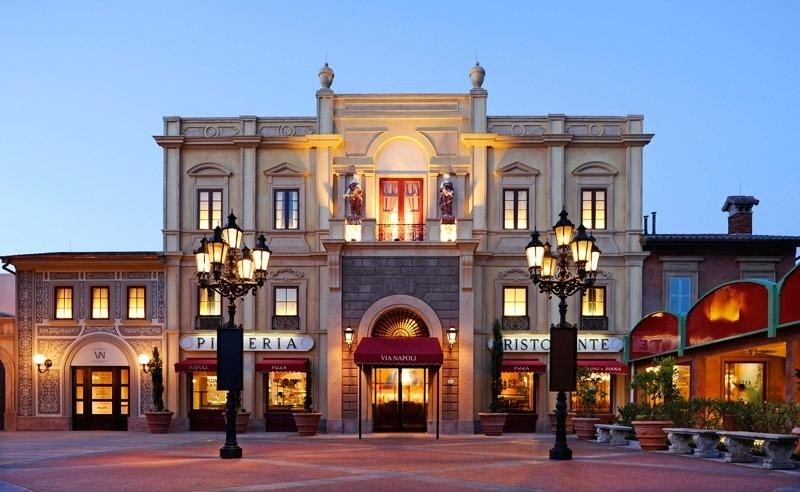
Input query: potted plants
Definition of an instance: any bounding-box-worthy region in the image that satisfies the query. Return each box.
[548,356,800,452]
[292,358,322,436]
[478,316,508,436]
[143,367,174,434]
[222,391,251,435]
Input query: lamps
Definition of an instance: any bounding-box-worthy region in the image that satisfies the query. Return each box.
[35,354,52,373]
[446,325,458,350]
[344,325,355,350]
[139,354,152,373]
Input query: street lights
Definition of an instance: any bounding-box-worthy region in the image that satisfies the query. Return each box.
[523,203,603,461]
[190,208,272,460]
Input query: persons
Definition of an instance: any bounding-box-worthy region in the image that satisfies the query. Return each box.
[438,173,456,223]
[343,174,364,224]
